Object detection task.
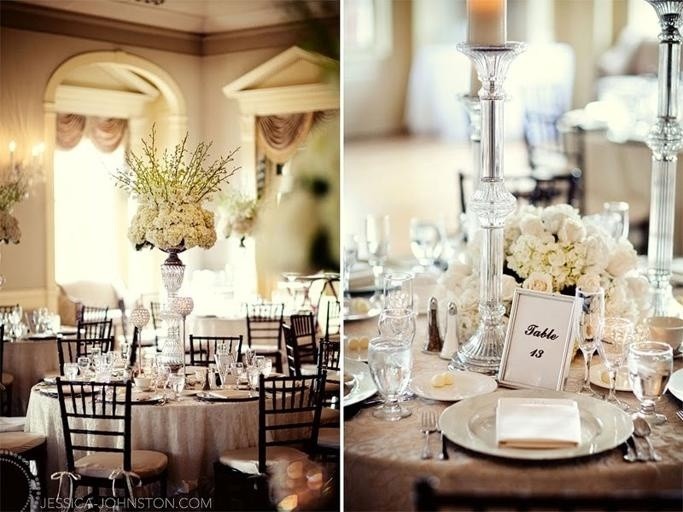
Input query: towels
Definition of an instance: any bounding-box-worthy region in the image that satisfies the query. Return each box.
[494,396,584,449]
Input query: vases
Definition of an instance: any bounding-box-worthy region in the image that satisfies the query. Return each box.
[156,240,187,298]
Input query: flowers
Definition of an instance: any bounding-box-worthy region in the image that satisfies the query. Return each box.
[0,183,29,244]
[109,120,243,249]
[216,192,274,238]
[250,118,339,272]
[459,204,651,326]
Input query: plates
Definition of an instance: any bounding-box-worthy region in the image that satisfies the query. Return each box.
[97,394,164,404]
[40,386,104,399]
[345,294,682,464]
[25,329,85,342]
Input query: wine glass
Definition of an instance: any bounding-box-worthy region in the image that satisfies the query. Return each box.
[64,344,273,401]
[345,192,630,295]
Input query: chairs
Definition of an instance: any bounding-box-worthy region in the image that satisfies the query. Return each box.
[452,164,576,211]
[409,478,683,512]
[520,108,567,172]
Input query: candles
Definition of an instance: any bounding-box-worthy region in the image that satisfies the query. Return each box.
[466,0,507,50]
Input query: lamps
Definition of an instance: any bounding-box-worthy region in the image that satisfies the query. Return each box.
[6,139,45,178]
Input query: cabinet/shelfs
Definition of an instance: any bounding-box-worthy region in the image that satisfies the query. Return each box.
[558,99,683,254]
[345,255,683,511]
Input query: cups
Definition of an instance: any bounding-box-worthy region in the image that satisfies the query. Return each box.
[0,303,63,341]
